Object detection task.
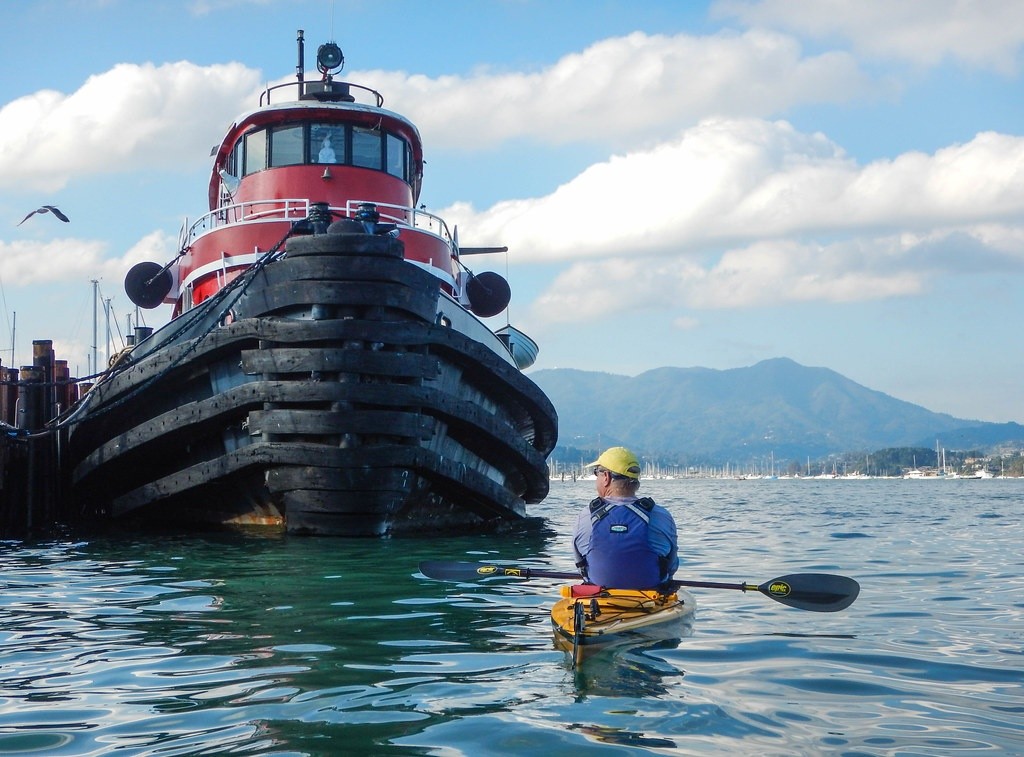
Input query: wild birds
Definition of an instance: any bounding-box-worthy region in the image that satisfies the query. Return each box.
[16,205,70,227]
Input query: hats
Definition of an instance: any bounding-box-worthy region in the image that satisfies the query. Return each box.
[583,447,640,481]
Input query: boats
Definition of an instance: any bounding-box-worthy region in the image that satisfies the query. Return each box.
[61,25,559,541]
[550,582,698,649]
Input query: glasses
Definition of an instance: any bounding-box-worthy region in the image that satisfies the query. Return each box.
[593,469,607,477]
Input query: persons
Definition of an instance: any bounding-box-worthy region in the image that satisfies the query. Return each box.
[571,446,680,591]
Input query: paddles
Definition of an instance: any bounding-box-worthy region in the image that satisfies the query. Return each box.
[418,558,862,613]
